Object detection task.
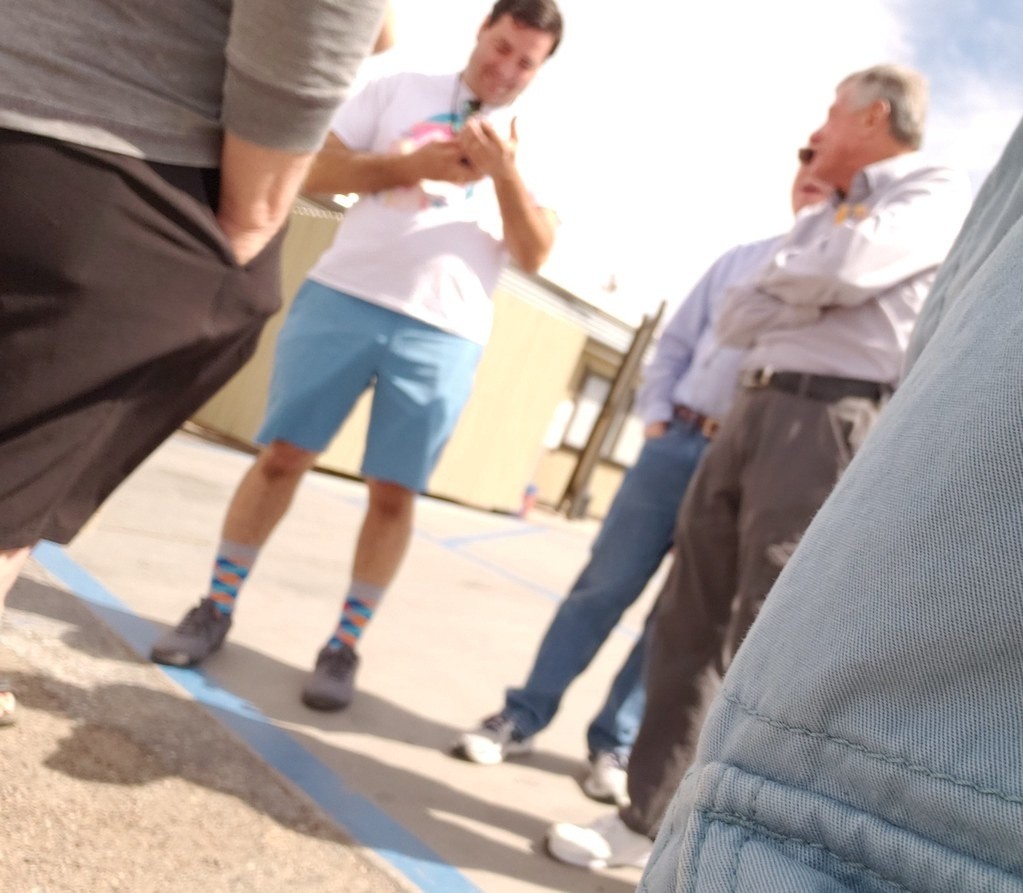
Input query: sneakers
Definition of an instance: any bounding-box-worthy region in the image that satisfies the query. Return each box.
[461,706,532,764]
[582,750,631,807]
[151,598,232,666]
[548,813,654,869]
[301,644,359,711]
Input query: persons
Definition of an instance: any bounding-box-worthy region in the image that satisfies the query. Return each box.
[147,1,563,711]
[545,64,962,869]
[637,123,1023,893]
[0,1,385,726]
[456,128,839,803]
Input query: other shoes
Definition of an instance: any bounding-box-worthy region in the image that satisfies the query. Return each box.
[0,690,17,725]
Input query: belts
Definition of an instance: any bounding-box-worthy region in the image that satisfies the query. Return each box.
[665,405,718,438]
[736,369,895,403]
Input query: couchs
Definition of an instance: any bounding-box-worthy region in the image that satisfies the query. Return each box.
[183,204,592,516]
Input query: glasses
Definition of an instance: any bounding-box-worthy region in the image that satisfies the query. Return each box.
[799,148,816,164]
